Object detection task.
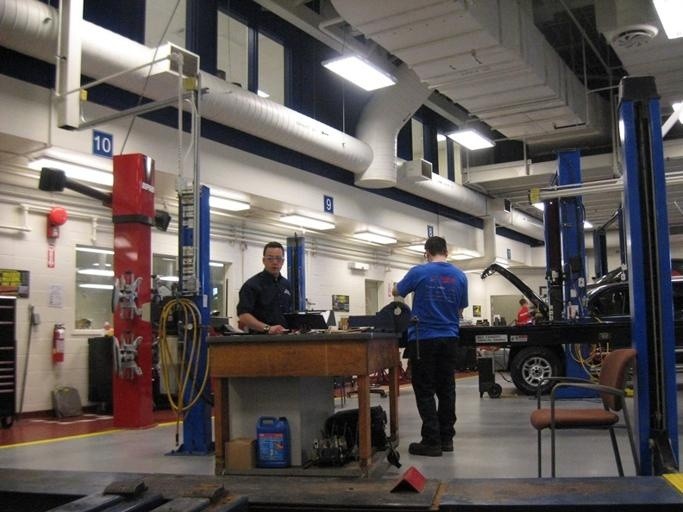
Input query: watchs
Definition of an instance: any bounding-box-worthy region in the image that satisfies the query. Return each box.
[263,323,272,333]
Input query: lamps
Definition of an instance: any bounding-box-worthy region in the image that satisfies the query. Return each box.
[190,181,253,213]
[445,128,495,151]
[25,154,114,187]
[354,227,400,249]
[321,52,399,92]
[672,101,683,125]
[280,211,337,233]
[454,246,512,271]
[652,0,682,40]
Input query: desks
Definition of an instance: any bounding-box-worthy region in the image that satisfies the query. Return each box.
[88,334,177,411]
[205,332,403,474]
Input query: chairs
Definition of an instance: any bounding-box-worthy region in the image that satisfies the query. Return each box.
[530,348,638,479]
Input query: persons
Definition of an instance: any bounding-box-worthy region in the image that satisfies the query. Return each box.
[391,237,468,455]
[517,298,530,324]
[235,241,297,334]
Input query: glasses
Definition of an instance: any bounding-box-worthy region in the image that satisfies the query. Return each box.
[263,255,284,263]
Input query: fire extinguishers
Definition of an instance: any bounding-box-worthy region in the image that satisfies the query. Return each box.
[52,324,66,363]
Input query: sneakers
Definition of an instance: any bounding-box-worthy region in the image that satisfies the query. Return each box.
[408,438,453,456]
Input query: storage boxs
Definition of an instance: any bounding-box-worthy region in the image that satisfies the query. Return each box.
[225,437,256,470]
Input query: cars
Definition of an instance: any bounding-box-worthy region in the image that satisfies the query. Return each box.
[480,264,683,384]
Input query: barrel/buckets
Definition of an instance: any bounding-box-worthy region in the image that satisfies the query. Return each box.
[256,416,291,468]
